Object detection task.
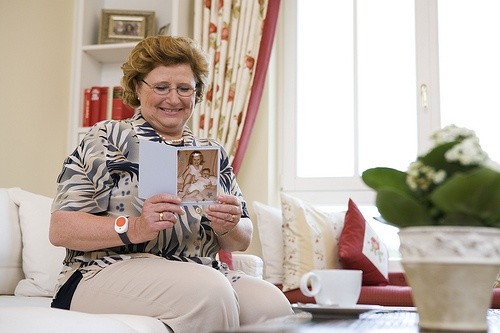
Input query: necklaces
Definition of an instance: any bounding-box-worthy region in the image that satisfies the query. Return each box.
[156,133,183,143]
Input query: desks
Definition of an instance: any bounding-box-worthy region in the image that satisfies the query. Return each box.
[212,310,500,333]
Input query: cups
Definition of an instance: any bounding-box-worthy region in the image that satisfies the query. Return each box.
[300,269,363,306]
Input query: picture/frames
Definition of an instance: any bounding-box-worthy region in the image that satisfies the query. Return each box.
[99,9,156,45]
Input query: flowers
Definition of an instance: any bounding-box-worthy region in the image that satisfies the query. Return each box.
[361,124,500,226]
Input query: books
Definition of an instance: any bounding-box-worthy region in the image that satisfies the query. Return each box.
[83,86,135,126]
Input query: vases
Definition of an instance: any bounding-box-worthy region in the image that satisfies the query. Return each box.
[398,227,500,333]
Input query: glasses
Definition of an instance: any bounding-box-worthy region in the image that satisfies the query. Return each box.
[139,79,197,96]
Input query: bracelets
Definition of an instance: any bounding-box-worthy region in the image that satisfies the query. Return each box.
[213,230,228,237]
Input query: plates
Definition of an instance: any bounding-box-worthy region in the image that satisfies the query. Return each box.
[292,303,380,319]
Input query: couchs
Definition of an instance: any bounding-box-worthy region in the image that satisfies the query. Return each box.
[0,187,264,333]
[276,259,500,309]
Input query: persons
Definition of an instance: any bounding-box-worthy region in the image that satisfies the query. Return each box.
[48,35,295,333]
[177,150,217,199]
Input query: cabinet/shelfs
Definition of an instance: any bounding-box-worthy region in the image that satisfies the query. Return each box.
[69,0,180,153]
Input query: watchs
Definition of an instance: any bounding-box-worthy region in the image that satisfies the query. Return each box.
[114,215,133,245]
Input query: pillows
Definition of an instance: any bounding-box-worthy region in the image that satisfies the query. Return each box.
[338,198,390,286]
[280,188,344,293]
[11,188,66,297]
[252,201,283,285]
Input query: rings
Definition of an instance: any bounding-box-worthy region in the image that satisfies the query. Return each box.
[160,213,163,220]
[231,214,236,221]
